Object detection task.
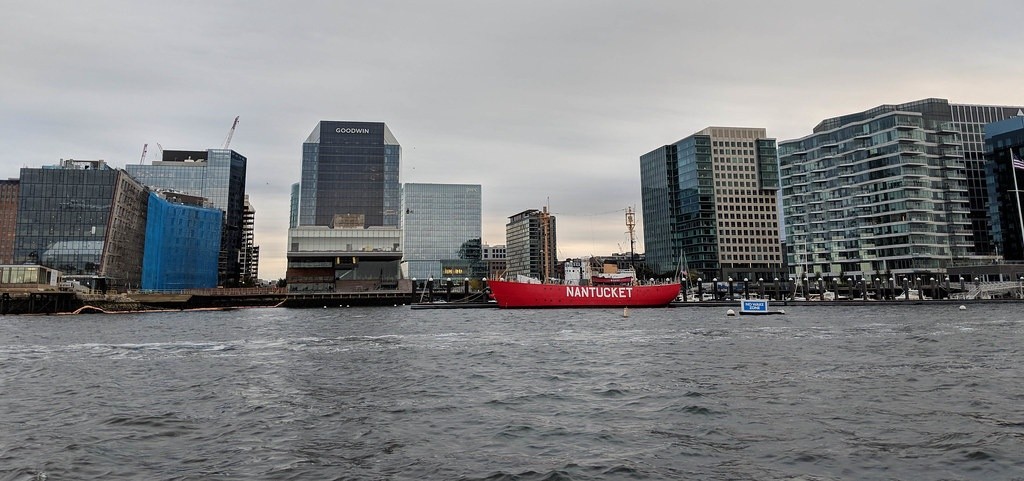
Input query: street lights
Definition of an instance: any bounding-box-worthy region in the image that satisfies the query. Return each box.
[945,277,950,294]
[729,278,734,300]
[681,278,687,301]
[744,278,749,300]
[464,278,469,302]
[875,278,881,300]
[861,278,867,300]
[697,278,703,301]
[930,278,935,299]
[412,278,416,303]
[803,278,810,301]
[447,278,451,302]
[903,278,909,300]
[833,278,838,301]
[975,277,979,300]
[847,278,853,300]
[774,278,780,300]
[482,277,487,302]
[666,278,670,284]
[713,278,718,301]
[917,278,922,299]
[759,278,764,299]
[429,278,433,303]
[960,277,965,292]
[789,278,794,300]
[889,278,895,300]
[818,278,824,300]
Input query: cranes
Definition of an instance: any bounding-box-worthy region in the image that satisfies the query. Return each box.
[220,116,239,149]
[156,142,163,156]
[139,144,148,164]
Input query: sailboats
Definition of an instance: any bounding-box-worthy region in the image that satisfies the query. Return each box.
[787,238,807,301]
[673,249,715,302]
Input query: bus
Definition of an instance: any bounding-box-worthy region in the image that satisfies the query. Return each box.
[693,294,715,301]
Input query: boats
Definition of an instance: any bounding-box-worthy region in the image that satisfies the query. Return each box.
[894,290,933,300]
[739,299,786,315]
[486,274,682,309]
[823,292,850,300]
